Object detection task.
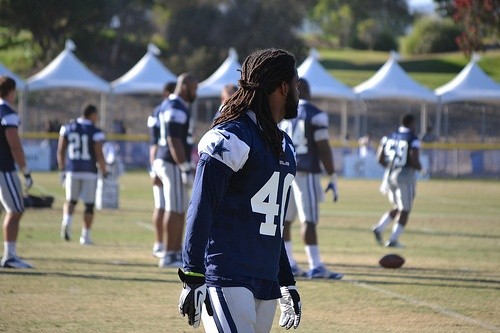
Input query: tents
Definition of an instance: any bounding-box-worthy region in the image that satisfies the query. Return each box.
[0,49,500,143]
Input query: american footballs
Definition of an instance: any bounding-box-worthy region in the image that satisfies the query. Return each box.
[379,255,405,268]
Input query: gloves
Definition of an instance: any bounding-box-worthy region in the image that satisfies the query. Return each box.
[179,162,195,186]
[278,286,301,330]
[325,172,339,201]
[23,169,33,191]
[178,268,215,329]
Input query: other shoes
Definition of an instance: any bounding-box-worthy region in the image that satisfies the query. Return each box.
[0,256,33,269]
[159,252,183,267]
[386,240,405,248]
[309,266,343,279]
[61,226,71,241]
[79,236,92,245]
[373,227,383,244]
[291,266,311,279]
[153,247,163,257]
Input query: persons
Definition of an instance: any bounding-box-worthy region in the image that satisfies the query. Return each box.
[371,112,423,249]
[0,75,33,268]
[146,72,198,269]
[179,47,305,333]
[280,78,344,281]
[58,104,109,244]
